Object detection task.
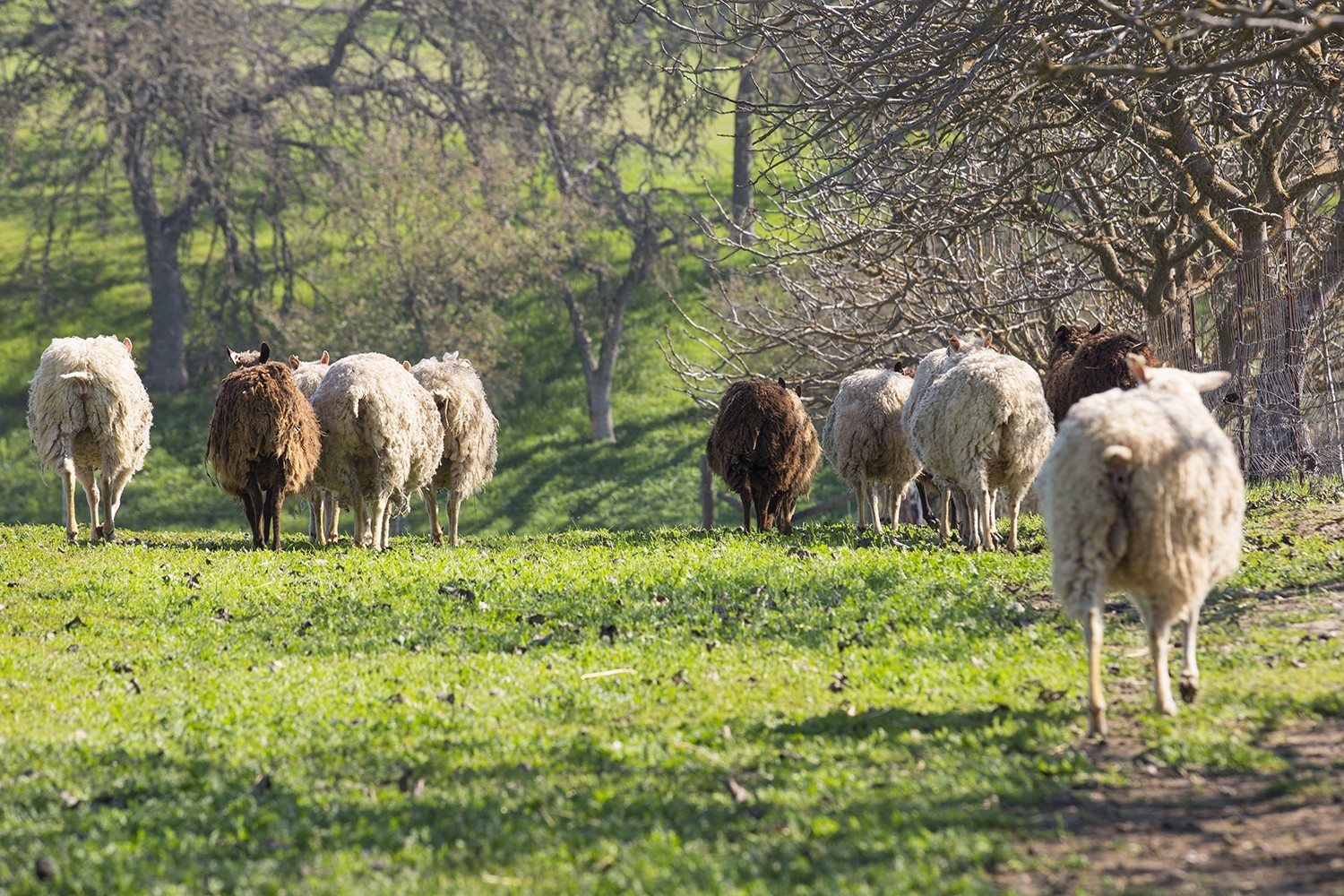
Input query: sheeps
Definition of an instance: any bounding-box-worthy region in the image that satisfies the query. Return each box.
[313,352,447,556]
[400,350,498,548]
[1033,351,1245,738]
[203,339,324,556]
[24,332,155,546]
[820,359,924,543]
[706,375,824,540]
[1035,324,1157,434]
[283,347,344,548]
[898,325,1056,554]
